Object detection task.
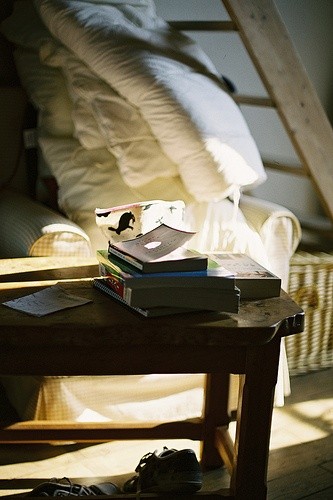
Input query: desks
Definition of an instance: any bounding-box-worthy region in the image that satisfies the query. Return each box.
[0,255,305,500]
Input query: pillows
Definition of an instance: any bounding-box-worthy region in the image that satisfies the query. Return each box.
[0,1,268,265]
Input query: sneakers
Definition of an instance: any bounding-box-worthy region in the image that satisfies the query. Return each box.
[120,446,203,495]
[31,477,123,496]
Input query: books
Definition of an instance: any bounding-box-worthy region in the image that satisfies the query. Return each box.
[203,253,282,302]
[91,223,240,318]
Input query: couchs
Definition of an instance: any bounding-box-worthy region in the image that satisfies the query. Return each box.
[0,85,302,447]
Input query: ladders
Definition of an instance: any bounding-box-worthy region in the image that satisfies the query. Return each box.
[155,0,333,255]
